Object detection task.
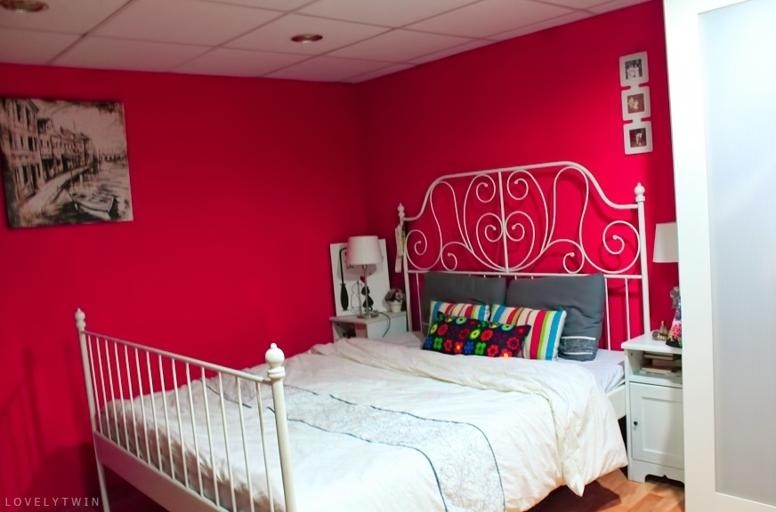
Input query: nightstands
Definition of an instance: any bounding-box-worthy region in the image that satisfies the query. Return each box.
[621,325,686,489]
[328,312,406,345]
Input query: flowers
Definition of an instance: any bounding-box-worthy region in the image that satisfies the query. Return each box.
[385,287,405,302]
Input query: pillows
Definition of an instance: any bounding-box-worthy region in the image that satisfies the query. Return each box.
[505,270,610,364]
[420,268,508,342]
[419,309,533,361]
[424,298,492,347]
[486,302,570,366]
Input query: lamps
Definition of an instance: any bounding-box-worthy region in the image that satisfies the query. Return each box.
[345,234,382,320]
[653,220,680,262]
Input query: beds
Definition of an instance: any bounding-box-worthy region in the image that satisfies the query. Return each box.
[70,155,663,511]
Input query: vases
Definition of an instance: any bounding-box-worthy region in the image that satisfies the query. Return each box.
[390,302,402,313]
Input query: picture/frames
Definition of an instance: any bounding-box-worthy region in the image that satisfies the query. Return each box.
[619,51,653,155]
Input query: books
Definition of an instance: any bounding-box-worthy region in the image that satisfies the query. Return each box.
[641,351,681,374]
